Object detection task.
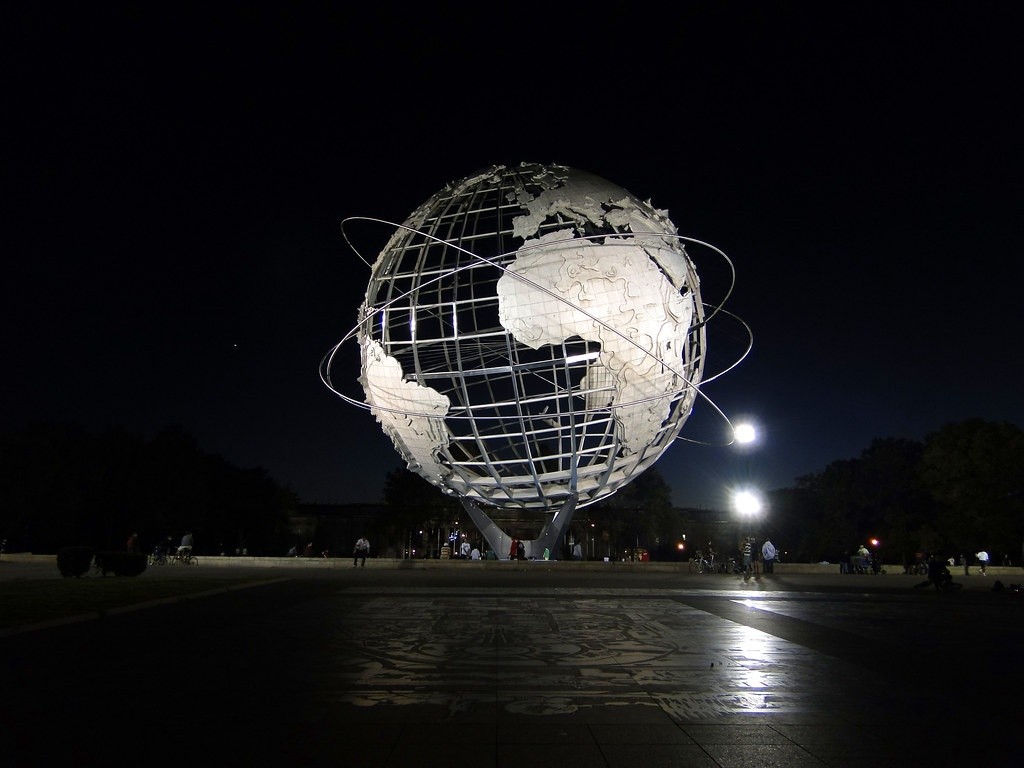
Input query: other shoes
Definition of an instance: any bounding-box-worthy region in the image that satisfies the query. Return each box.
[353,565,356,568]
[980,569,982,573]
[700,571,703,573]
[983,573,986,576]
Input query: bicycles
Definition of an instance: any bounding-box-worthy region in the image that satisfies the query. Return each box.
[172,549,199,567]
[149,550,171,566]
[689,552,736,576]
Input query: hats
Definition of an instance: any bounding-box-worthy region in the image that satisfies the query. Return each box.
[444,543,448,546]
[859,545,864,547]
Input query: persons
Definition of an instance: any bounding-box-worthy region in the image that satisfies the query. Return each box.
[507,536,525,560]
[1002,554,1011,567]
[903,549,989,576]
[441,543,451,559]
[353,535,370,566]
[839,541,882,574]
[288,541,329,559]
[542,547,549,561]
[738,536,781,575]
[461,538,480,560]
[573,542,582,561]
[126,530,193,560]
[928,552,953,588]
[221,548,246,557]
[703,539,716,567]
